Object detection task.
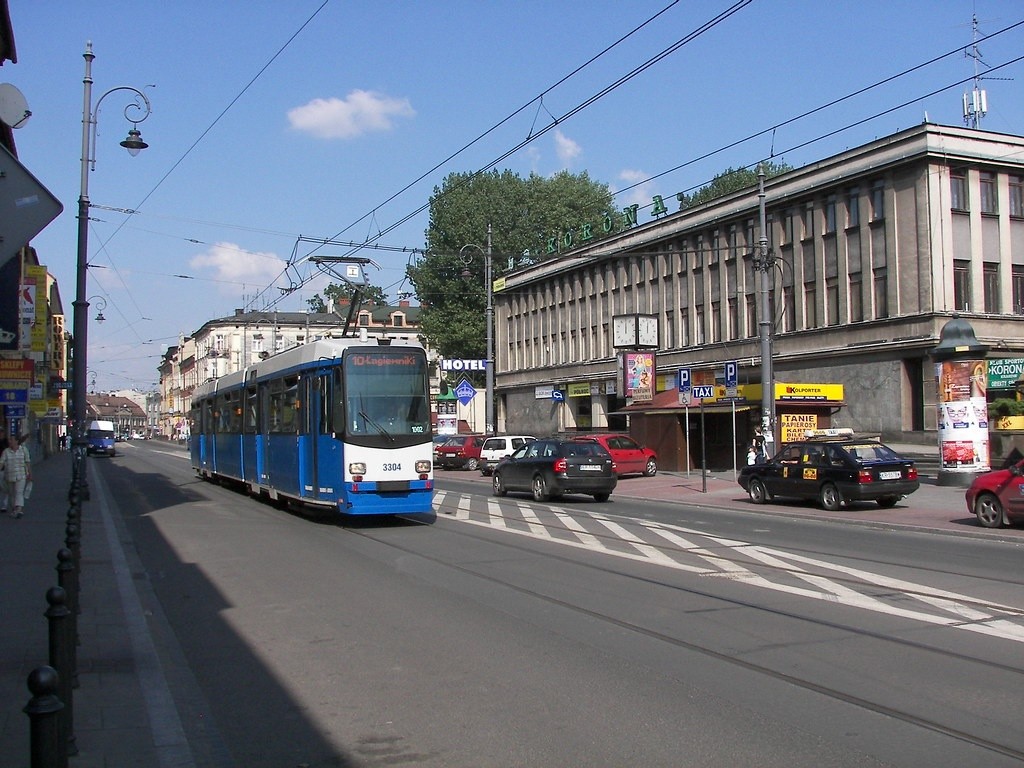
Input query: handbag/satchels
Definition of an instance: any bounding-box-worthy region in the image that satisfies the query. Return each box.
[24,480,32,499]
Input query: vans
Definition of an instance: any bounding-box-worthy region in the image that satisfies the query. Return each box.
[86,421,116,457]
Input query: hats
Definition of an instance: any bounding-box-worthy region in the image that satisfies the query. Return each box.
[0,427,5,431]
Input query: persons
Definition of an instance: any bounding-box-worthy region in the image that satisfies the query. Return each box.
[0,434,32,519]
[61,433,72,452]
[747,424,771,466]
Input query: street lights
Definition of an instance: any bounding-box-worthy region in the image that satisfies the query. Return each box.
[745,158,776,450]
[68,35,156,498]
[459,223,495,435]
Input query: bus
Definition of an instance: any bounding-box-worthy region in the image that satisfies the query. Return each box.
[187,256,451,527]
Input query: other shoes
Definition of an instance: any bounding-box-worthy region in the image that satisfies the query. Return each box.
[0,505,8,511]
[10,509,24,519]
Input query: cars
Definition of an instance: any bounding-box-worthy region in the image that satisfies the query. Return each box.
[737,428,919,512]
[433,433,454,468]
[489,436,618,503]
[569,432,658,479]
[436,434,495,472]
[480,436,541,477]
[964,457,1024,527]
